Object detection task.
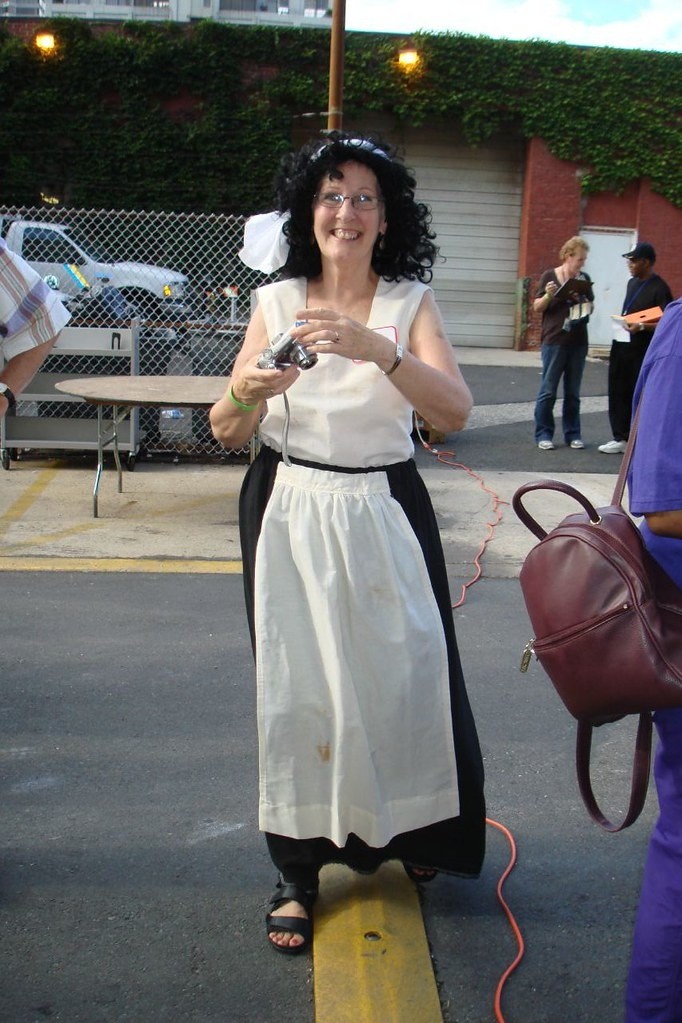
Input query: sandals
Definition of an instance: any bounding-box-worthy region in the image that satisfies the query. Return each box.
[265,872,320,954]
[403,864,438,883]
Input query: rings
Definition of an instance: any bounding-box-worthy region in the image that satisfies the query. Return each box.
[335,331,340,342]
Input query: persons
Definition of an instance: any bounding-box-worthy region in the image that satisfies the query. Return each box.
[531,237,594,449]
[0,238,73,423]
[210,132,487,954]
[597,242,672,453]
[622,299,682,1023]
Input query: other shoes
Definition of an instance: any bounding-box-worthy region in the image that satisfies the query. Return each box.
[598,440,628,454]
[571,440,584,448]
[538,440,554,449]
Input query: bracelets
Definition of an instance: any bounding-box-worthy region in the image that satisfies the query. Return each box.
[639,322,645,330]
[543,293,553,302]
[381,344,404,375]
[229,385,260,411]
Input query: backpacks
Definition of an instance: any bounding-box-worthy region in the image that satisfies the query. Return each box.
[512,395,682,832]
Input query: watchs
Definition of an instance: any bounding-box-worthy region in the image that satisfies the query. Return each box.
[0,383,16,409]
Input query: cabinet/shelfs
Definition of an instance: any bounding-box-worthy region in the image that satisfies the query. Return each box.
[1,316,147,472]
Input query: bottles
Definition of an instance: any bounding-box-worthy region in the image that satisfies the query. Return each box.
[162,410,184,418]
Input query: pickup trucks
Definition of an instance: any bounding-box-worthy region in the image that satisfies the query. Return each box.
[0,213,194,324]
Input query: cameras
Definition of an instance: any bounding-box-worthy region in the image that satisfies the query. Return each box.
[255,322,318,371]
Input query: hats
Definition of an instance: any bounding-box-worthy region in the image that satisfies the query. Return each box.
[621,242,656,262]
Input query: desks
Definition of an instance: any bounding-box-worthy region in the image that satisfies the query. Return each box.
[55,374,258,517]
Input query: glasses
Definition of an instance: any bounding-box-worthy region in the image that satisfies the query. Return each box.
[313,192,385,210]
[625,259,640,265]
